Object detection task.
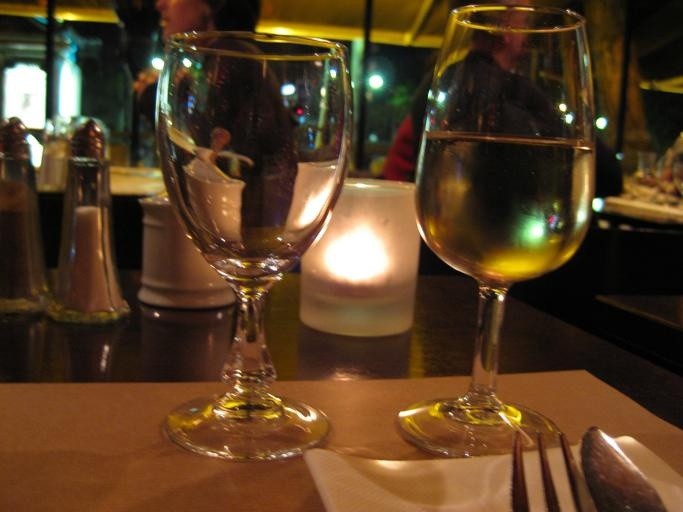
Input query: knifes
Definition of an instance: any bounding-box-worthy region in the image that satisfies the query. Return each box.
[580,426,667,511]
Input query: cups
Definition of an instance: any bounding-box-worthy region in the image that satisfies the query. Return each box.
[138,188,239,309]
[299,177,422,339]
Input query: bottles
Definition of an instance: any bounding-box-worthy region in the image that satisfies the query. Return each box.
[39,117,132,321]
[628,150,657,188]
[1,116,53,315]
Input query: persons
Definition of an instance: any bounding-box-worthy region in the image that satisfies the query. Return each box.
[130,1,301,257]
[410,0,626,334]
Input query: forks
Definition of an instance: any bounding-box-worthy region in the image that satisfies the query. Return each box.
[508,429,598,511]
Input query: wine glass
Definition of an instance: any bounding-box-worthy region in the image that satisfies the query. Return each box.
[155,29,353,462]
[655,160,683,210]
[395,4,596,458]
